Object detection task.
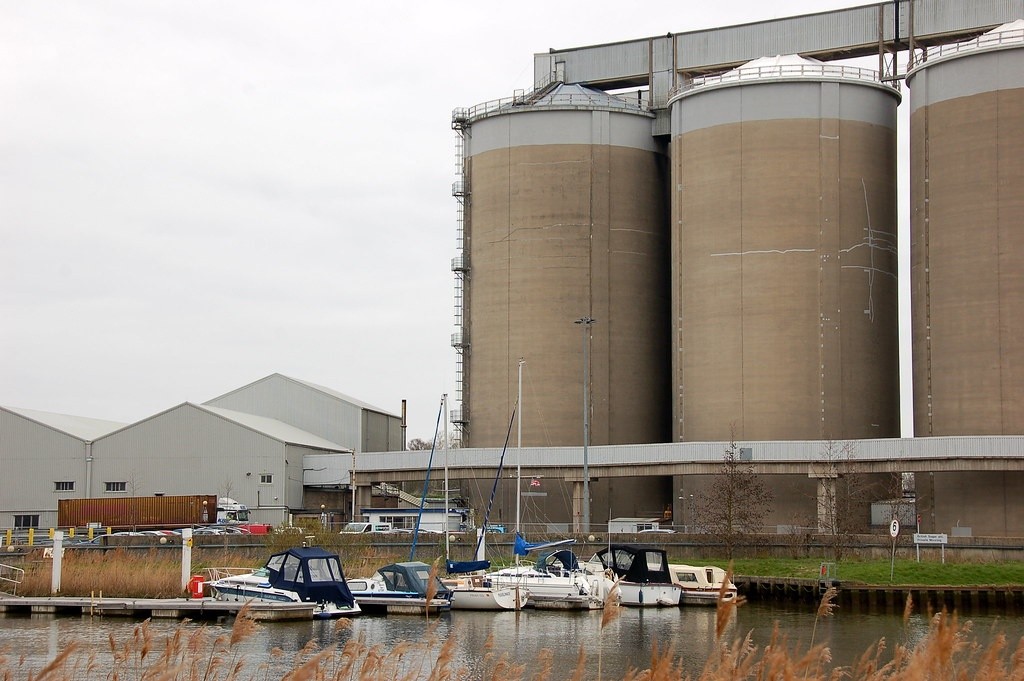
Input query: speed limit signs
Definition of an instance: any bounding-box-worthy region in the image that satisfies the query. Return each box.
[889,520,901,537]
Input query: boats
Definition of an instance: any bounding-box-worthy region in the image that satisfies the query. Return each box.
[202,541,363,620]
[346,561,456,615]
[648,562,737,607]
[596,543,682,609]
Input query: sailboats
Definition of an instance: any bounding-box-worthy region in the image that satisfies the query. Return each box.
[408,360,622,611]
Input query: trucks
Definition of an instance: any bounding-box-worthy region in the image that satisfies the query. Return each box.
[237,524,274,535]
[216,497,252,525]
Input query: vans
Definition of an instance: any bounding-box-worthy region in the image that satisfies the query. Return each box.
[339,522,392,534]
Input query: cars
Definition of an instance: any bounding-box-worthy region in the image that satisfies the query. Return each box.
[638,529,679,533]
[75,523,252,545]
[486,525,507,534]
[274,526,303,535]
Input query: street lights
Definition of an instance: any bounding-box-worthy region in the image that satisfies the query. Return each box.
[575,313,599,533]
